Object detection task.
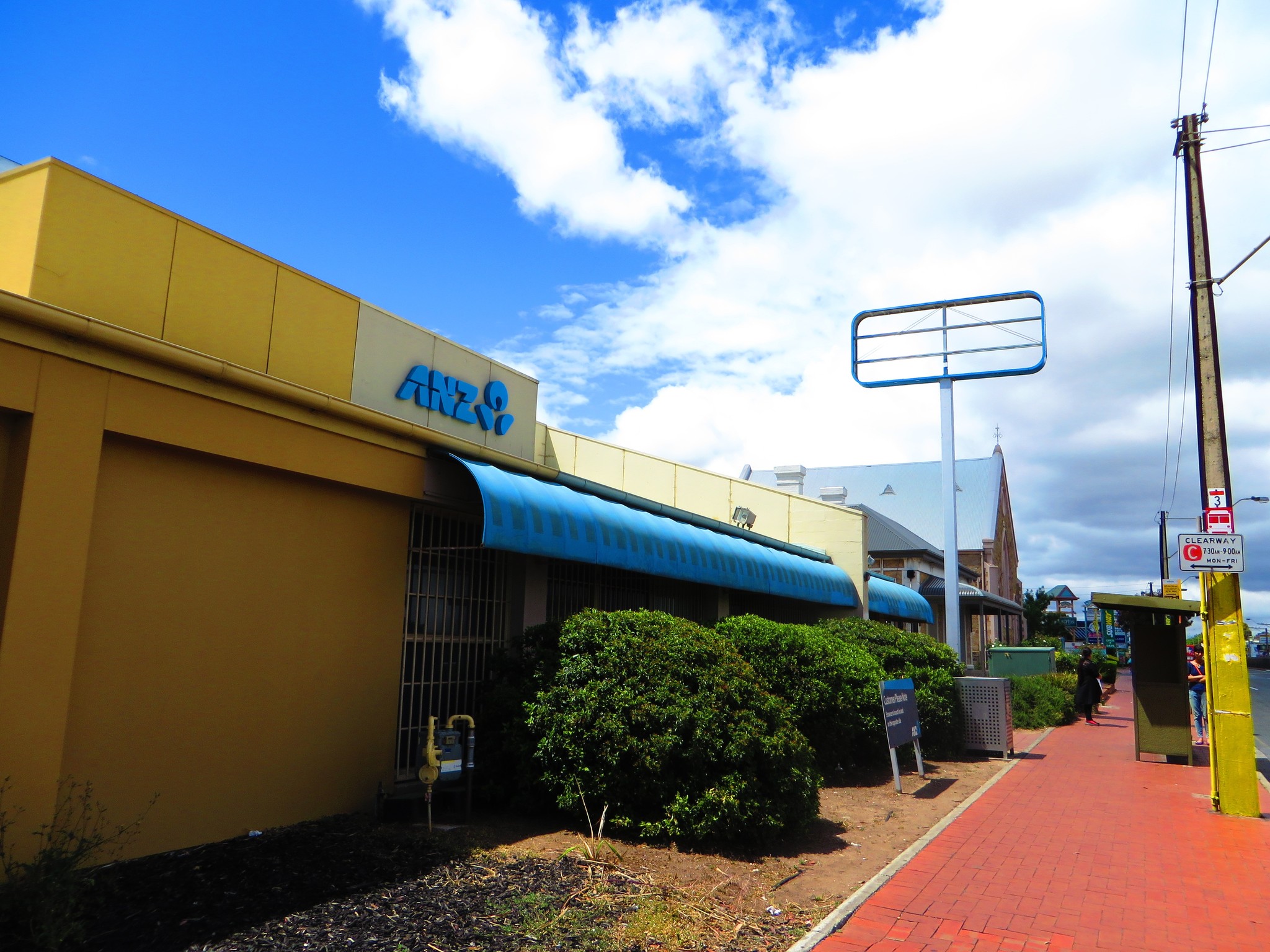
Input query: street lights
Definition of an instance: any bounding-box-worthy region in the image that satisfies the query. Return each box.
[1158,495,1270,597]
[1246,617,1269,658]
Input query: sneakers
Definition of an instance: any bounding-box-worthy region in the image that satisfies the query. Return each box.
[1085,719,1099,726]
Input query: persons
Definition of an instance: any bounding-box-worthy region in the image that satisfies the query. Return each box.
[1187,644,1212,745]
[1075,646,1103,726]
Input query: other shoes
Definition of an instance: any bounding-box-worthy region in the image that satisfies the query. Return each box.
[1206,742,1209,745]
[1195,741,1204,744]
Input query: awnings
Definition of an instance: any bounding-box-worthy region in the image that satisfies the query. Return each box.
[867,570,933,625]
[446,452,859,608]
